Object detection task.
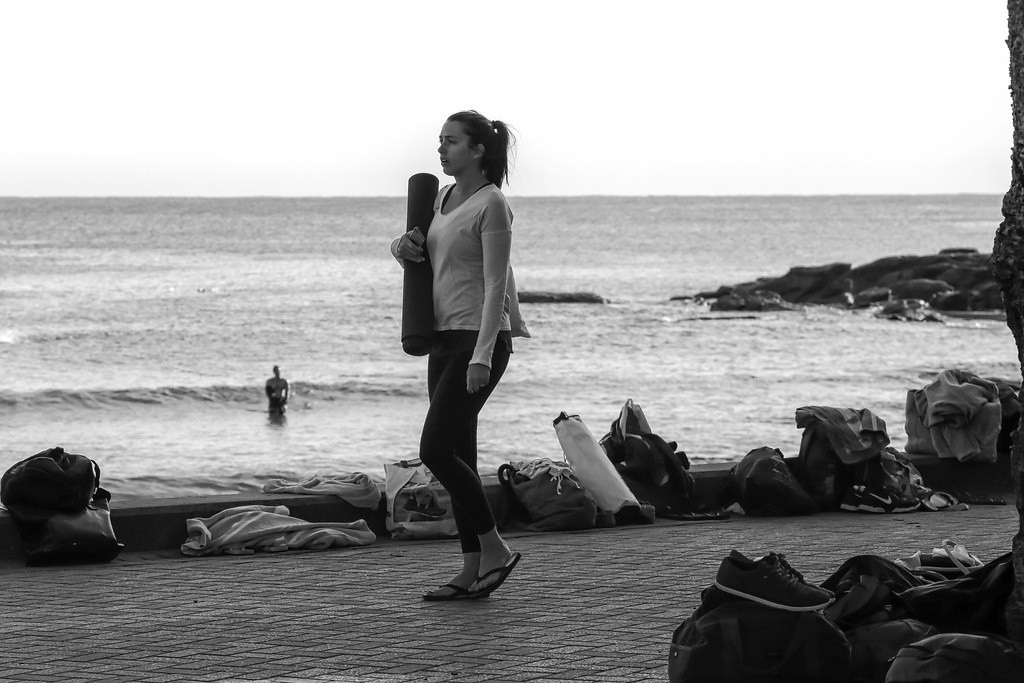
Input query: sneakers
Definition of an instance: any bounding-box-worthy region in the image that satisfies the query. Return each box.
[840,485,921,513]
[713,548,836,612]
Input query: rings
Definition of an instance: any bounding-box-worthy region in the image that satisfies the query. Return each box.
[480,384,485,386]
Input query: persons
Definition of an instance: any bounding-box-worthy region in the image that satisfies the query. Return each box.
[390,109,520,602]
[265,364,289,415]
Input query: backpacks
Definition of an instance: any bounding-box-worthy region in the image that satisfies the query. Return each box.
[885,633,1004,683]
[496,461,597,531]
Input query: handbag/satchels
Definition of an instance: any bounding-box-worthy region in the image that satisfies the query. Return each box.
[730,447,821,515]
[668,587,851,683]
[1,447,100,511]
[385,458,459,541]
[4,497,125,567]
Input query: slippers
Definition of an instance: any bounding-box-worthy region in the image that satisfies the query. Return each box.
[422,583,468,601]
[468,552,521,598]
